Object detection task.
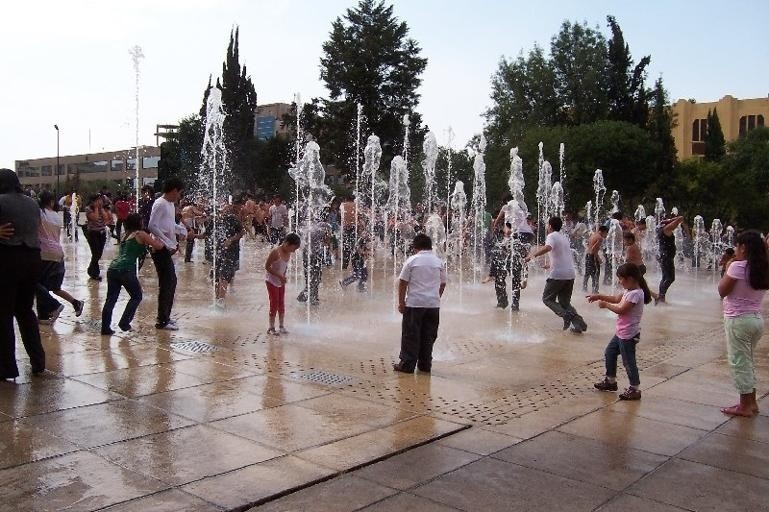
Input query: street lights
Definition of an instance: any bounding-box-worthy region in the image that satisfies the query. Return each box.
[53,124,60,200]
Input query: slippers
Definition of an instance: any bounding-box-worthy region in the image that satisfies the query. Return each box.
[720,405,753,417]
[735,403,760,413]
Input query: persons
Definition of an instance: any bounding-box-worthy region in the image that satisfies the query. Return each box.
[1,169,769,418]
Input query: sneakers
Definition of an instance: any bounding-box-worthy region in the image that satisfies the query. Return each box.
[562,310,575,330]
[216,298,225,307]
[310,296,319,305]
[102,329,115,334]
[155,323,179,330]
[594,377,618,392]
[417,364,431,372]
[51,304,64,322]
[619,385,641,400]
[339,280,347,291]
[118,323,131,329]
[75,301,84,317]
[357,287,367,292]
[168,318,177,323]
[267,327,279,335]
[393,364,414,373]
[570,321,587,332]
[296,292,308,303]
[278,326,288,334]
